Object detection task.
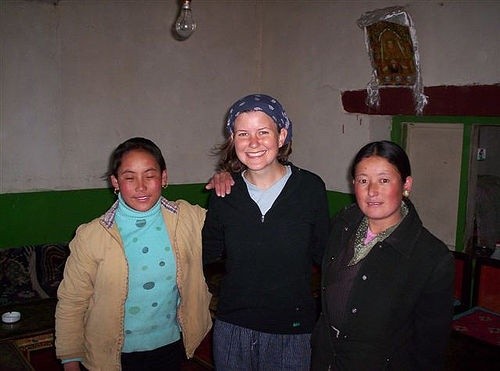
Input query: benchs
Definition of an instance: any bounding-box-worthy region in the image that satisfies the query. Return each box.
[0,183,356,299]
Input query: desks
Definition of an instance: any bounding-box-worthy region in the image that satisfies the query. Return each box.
[0,299,55,371]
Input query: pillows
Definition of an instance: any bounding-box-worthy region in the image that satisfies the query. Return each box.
[0,244,67,299]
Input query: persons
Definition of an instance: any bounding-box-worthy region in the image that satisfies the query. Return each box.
[55,137,235,371]
[309,140,456,371]
[201,94,330,371]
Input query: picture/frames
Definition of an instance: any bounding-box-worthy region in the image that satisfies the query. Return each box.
[361,11,418,88]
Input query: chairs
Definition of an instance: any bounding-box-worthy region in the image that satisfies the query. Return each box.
[448,251,500,371]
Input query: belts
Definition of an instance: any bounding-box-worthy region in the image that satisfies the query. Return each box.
[328,324,341,339]
